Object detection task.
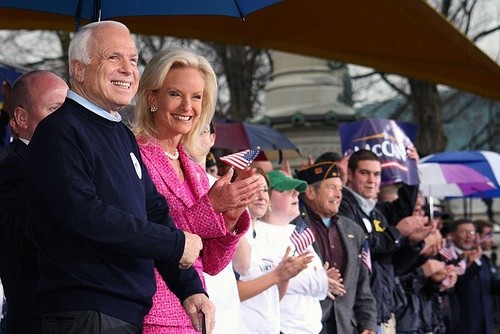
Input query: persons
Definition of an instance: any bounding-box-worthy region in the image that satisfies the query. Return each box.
[124,46,266,334]
[178,118,253,334]
[0,69,71,215]
[301,154,314,171]
[233,169,315,334]
[0,20,215,334]
[264,170,346,334]
[205,152,221,179]
[294,160,377,334]
[469,219,500,317]
[433,219,500,334]
[251,149,294,180]
[394,260,459,334]
[337,148,434,334]
[309,144,418,229]
[377,180,461,259]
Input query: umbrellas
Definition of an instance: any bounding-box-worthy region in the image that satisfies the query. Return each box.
[0,0,284,21]
[415,163,496,228]
[415,150,500,218]
[207,120,304,161]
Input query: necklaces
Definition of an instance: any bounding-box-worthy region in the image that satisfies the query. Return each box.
[162,147,180,162]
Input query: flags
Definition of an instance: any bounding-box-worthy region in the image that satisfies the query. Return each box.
[359,238,372,274]
[290,217,315,256]
[439,237,459,262]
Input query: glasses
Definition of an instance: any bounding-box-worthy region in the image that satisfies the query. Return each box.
[455,229,476,235]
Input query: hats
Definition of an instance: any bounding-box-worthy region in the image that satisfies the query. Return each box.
[205,153,216,168]
[266,170,308,193]
[297,162,343,185]
